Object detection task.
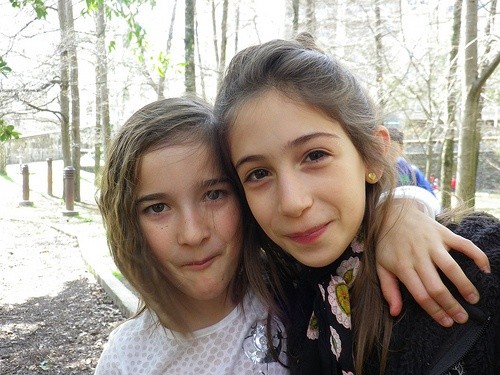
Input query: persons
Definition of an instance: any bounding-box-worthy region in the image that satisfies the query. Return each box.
[94,96,490,375]
[211,32,500,375]
[429,173,456,192]
[387,127,436,198]
